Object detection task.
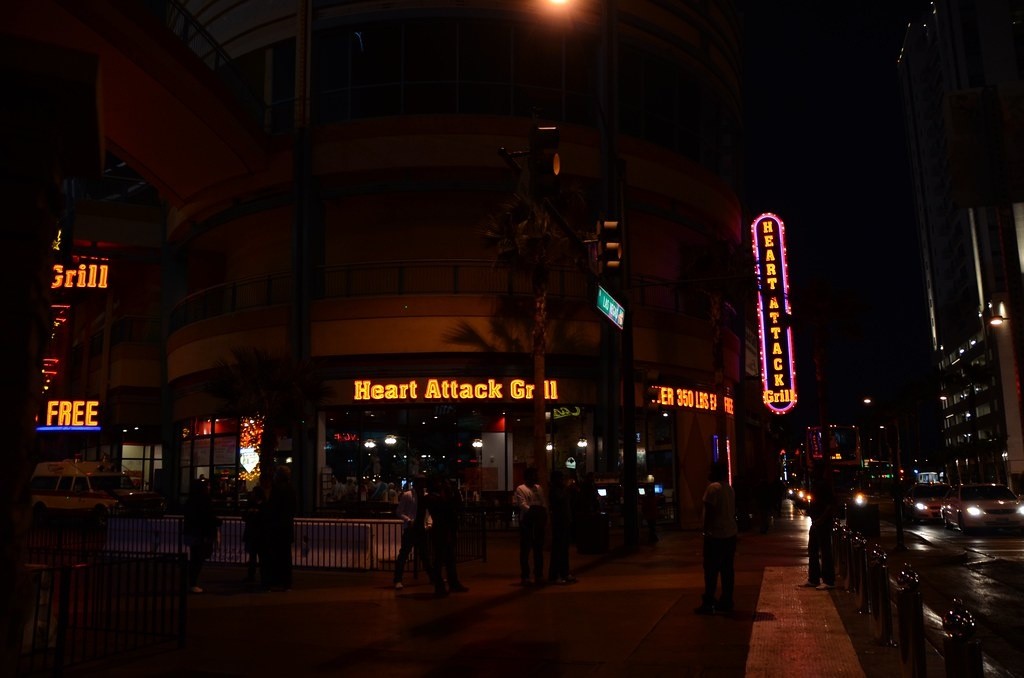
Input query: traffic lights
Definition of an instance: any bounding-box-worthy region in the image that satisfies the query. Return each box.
[596,220,623,274]
[529,124,563,196]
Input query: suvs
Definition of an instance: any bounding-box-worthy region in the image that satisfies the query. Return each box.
[29,453,166,531]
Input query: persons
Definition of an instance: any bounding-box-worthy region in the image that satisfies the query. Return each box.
[514,467,547,587]
[427,456,467,598]
[240,466,299,591]
[183,478,224,594]
[582,472,600,513]
[693,462,738,615]
[549,471,576,585]
[394,474,433,589]
[641,484,659,544]
[798,462,841,590]
[332,473,399,502]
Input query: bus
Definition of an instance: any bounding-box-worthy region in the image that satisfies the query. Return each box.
[833,459,869,510]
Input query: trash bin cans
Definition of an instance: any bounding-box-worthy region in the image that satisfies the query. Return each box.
[571,511,609,553]
[846,503,881,536]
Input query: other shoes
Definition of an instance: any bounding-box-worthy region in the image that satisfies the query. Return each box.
[799,581,834,591]
[448,583,468,592]
[716,600,736,614]
[191,586,205,593]
[519,577,544,588]
[545,574,577,584]
[394,582,404,589]
[693,604,717,615]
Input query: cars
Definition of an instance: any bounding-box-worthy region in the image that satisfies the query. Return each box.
[902,484,953,525]
[939,483,1024,536]
